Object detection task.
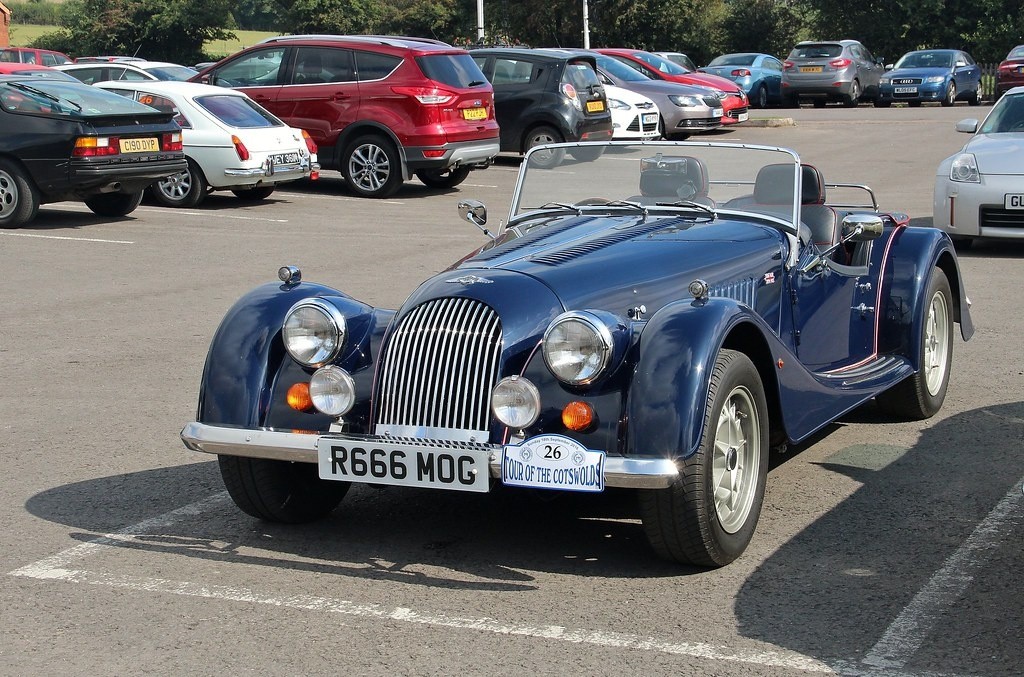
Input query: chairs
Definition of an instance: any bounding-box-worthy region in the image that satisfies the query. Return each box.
[626,156,715,208]
[513,61,533,83]
[300,54,326,84]
[806,48,821,58]
[823,47,837,56]
[723,163,842,250]
[494,65,510,84]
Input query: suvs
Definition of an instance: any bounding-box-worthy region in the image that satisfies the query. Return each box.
[635,52,695,78]
[186,33,499,198]
[777,39,887,107]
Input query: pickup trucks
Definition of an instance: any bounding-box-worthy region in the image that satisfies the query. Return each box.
[0,48,73,75]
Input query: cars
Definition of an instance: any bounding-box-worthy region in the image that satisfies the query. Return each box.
[468,48,615,170]
[933,84,1024,240]
[87,80,320,209]
[178,142,974,566]
[0,75,187,231]
[994,44,1024,102]
[0,62,90,112]
[591,47,751,127]
[569,51,725,141]
[696,53,785,109]
[878,50,983,107]
[484,59,661,153]
[50,56,333,87]
